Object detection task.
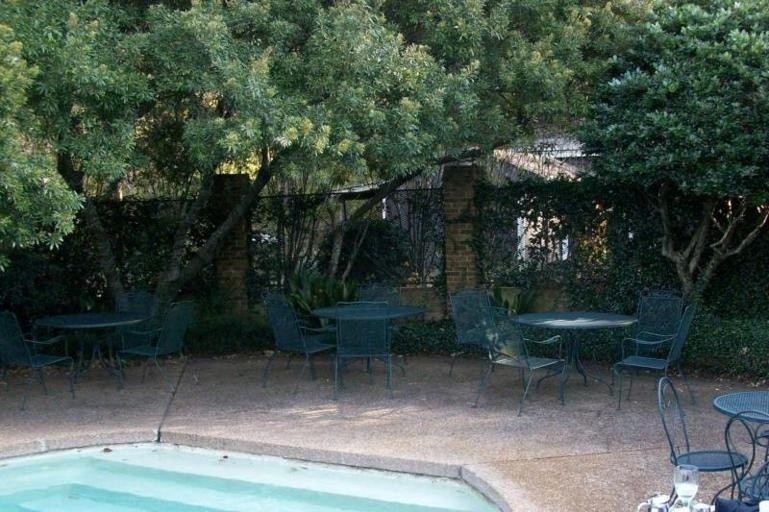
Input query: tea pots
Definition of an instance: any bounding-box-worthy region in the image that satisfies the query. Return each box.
[636,493,671,512]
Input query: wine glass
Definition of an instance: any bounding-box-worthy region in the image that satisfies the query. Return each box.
[673,465,700,512]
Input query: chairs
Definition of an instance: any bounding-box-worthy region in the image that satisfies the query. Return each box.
[2,285,199,411]
[253,288,429,390]
[655,375,769,510]
[445,284,696,417]
